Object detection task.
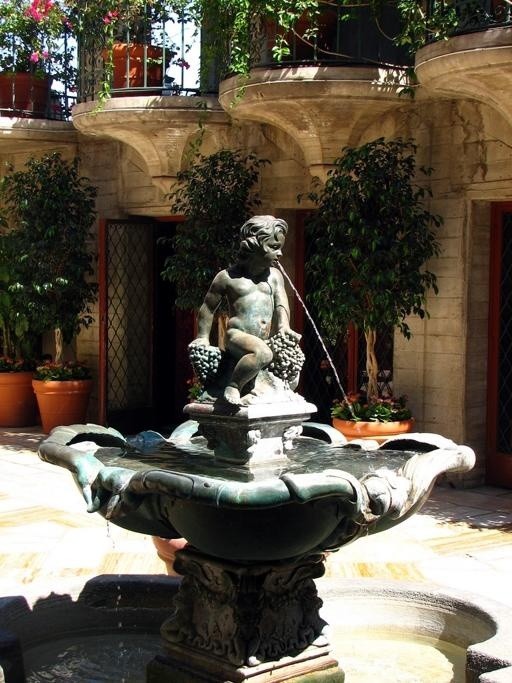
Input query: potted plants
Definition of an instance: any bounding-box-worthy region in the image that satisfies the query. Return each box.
[297,134,447,448]
[1,148,101,435]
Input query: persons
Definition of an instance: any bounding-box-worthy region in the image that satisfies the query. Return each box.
[186,214,306,406]
[311,354,342,423]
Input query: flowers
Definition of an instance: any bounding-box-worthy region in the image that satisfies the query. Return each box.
[1,0,80,91]
[81,0,189,118]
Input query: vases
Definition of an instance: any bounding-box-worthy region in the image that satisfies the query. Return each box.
[1,72,55,117]
[261,7,342,61]
[101,43,177,96]
